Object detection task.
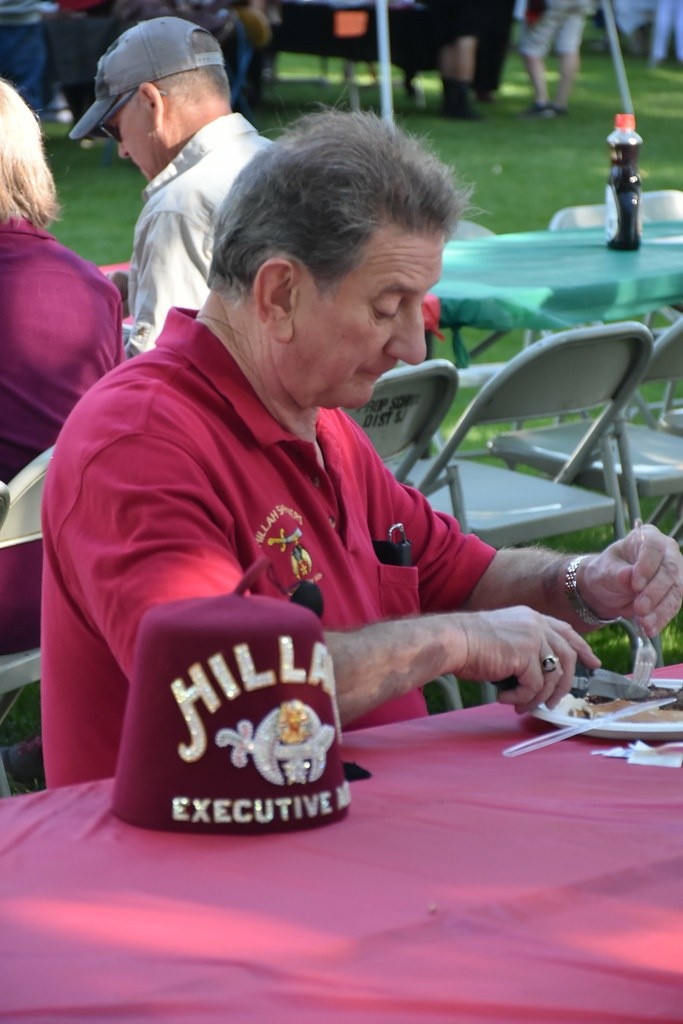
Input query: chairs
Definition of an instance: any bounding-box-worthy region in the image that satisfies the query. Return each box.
[0,192,683,801]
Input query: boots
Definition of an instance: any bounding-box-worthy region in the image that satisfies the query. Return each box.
[441,79,485,118]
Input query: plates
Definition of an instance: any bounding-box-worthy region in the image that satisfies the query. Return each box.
[531,678,683,743]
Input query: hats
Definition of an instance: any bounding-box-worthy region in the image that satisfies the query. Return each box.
[109,555,352,832]
[69,16,224,139]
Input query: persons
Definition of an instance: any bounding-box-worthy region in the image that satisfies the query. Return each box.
[0,16,275,786]
[41,105,683,789]
[0,0,602,116]
[0,82,133,656]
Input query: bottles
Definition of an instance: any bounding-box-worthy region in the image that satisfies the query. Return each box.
[605,115,641,252]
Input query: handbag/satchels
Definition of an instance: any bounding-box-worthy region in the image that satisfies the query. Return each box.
[513,0,545,23]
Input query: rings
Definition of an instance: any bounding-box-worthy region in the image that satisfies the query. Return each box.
[539,656,560,673]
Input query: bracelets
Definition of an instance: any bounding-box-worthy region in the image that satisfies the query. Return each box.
[565,554,623,625]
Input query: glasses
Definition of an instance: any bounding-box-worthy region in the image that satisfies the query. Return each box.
[97,87,167,142]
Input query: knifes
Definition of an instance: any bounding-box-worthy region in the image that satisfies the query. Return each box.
[502,698,677,758]
[497,669,652,705]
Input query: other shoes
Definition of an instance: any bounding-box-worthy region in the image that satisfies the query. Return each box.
[520,102,566,119]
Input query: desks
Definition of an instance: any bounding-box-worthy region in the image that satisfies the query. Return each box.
[246,0,520,115]
[0,663,683,1024]
[44,15,265,167]
[429,218,682,472]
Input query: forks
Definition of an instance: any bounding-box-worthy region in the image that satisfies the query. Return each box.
[632,520,655,685]
